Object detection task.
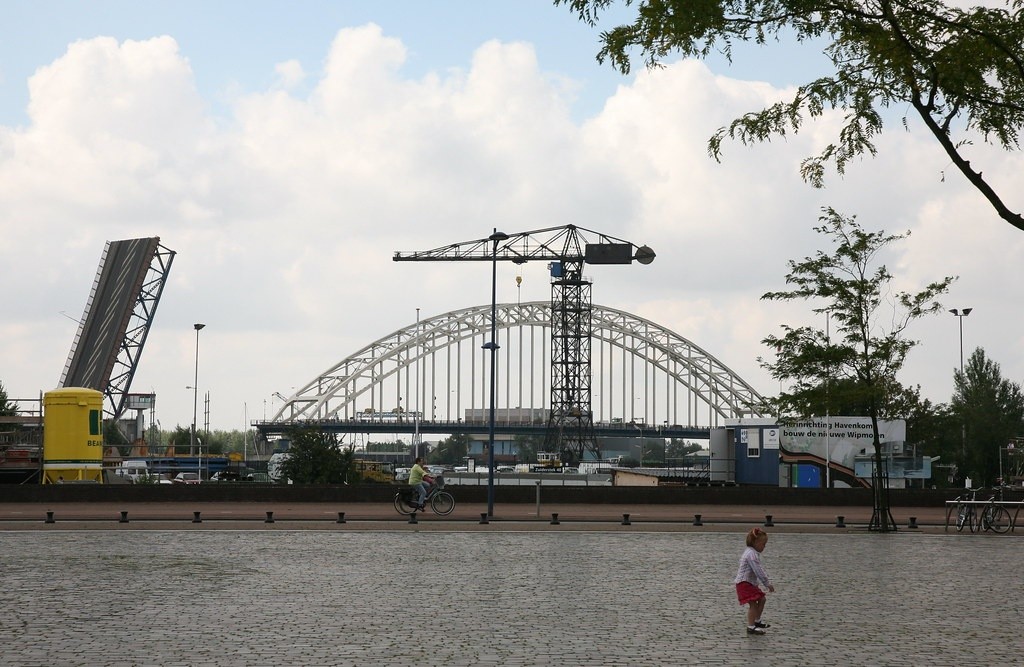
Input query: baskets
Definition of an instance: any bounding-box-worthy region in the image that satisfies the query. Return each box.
[959,491,973,500]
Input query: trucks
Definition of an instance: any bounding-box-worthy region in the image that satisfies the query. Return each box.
[116,460,151,485]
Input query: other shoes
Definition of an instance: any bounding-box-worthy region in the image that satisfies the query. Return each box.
[754,621,771,628]
[417,504,425,512]
[747,626,766,635]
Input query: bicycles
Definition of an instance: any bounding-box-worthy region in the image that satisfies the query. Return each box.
[392,481,456,516]
[955,486,984,533]
[977,478,1012,533]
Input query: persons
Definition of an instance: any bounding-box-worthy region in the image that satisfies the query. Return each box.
[734,528,776,635]
[409,457,435,512]
[1007,442,1014,471]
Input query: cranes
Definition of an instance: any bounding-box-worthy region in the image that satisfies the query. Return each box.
[390,224,658,468]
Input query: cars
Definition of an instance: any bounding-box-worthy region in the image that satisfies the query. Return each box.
[150,473,172,484]
[247,473,275,483]
[210,470,241,482]
[172,472,205,485]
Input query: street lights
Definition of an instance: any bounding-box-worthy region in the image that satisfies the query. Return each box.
[633,424,642,467]
[191,324,207,457]
[948,308,975,469]
[663,420,668,467]
[460,434,469,467]
[292,386,298,424]
[481,229,509,517]
[197,438,202,484]
[156,419,162,484]
